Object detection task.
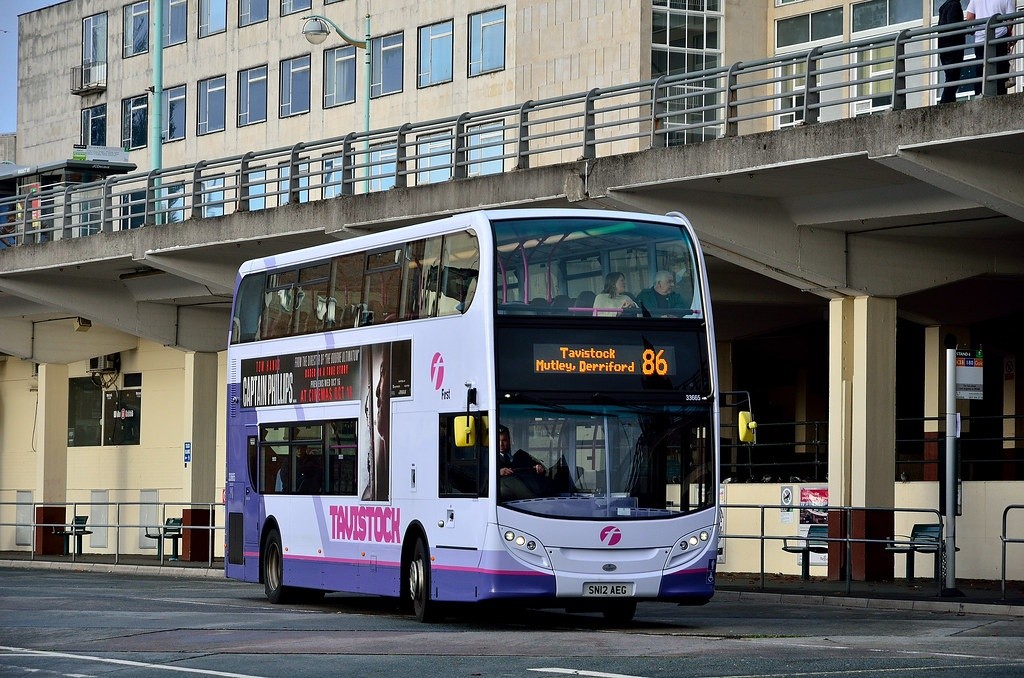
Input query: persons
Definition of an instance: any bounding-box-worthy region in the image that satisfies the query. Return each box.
[938,0,966,104]
[362,344,389,501]
[0,206,15,247]
[499,424,546,498]
[593,272,643,317]
[280,445,322,494]
[967,0,1016,97]
[635,270,693,318]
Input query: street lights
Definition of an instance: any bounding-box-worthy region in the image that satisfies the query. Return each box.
[302,13,369,194]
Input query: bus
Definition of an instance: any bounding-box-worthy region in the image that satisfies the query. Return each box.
[225,208,758,623]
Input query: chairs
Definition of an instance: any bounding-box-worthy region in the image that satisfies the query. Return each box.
[886,524,960,590]
[52,516,93,556]
[783,525,828,580]
[531,289,651,316]
[270,301,383,337]
[145,518,183,561]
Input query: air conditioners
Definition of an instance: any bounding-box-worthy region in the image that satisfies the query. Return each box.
[84,352,115,373]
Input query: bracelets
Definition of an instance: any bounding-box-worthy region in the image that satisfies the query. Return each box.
[7,242,11,247]
